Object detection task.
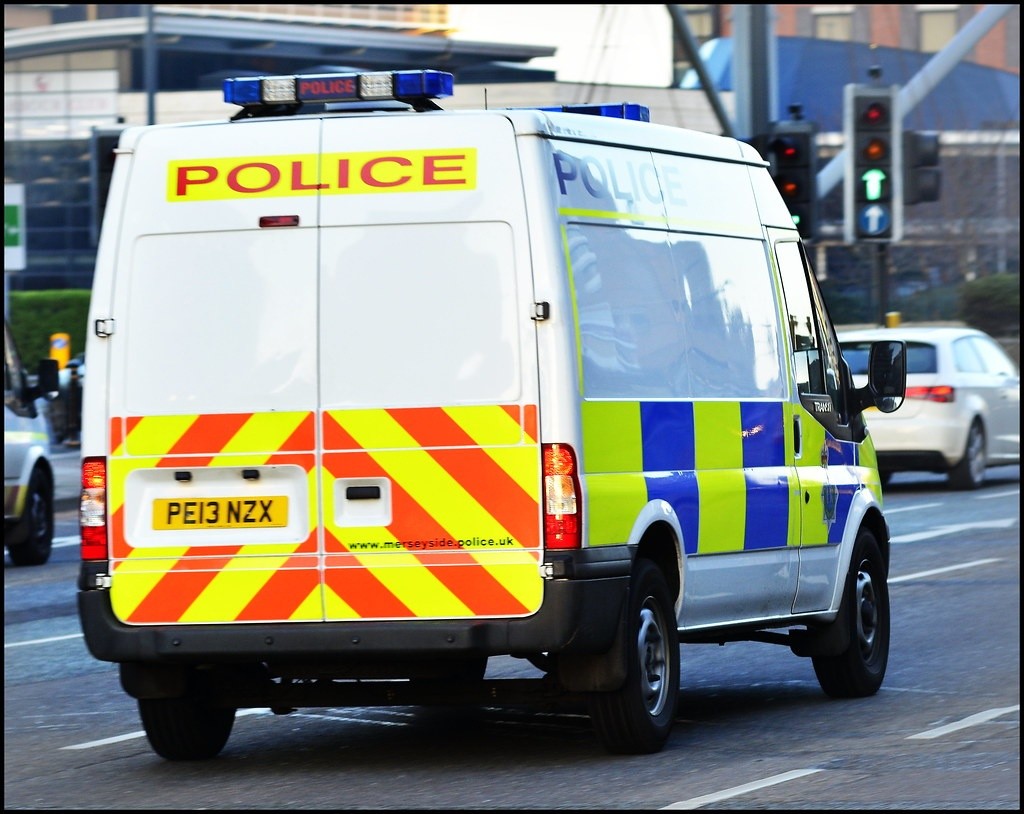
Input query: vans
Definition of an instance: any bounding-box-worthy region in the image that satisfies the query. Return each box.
[75,71,891,761]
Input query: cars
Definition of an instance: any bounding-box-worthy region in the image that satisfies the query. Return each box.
[836,327,1020,488]
[5,320,60,565]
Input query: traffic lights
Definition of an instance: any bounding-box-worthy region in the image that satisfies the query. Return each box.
[768,121,815,236]
[844,84,904,246]
[902,130,943,204]
